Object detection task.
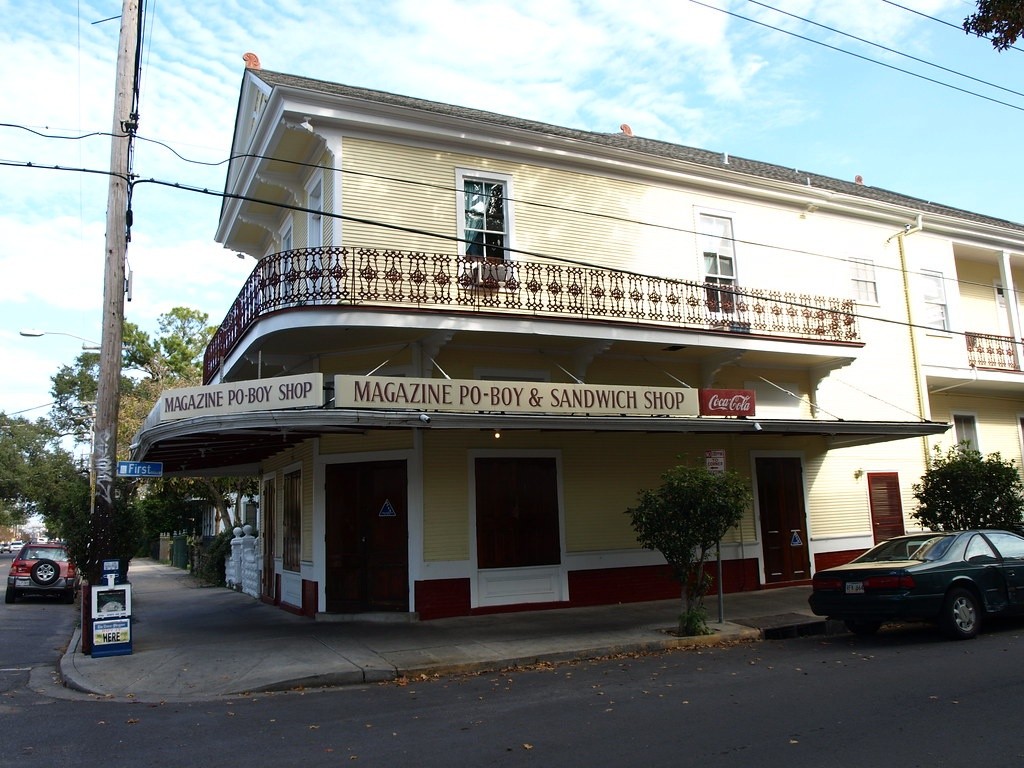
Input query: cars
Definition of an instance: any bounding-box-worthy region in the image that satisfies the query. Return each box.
[807,530,1024,641]
[0,542,12,555]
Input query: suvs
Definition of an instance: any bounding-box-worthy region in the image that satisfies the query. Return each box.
[9,542,23,553]
[4,541,81,605]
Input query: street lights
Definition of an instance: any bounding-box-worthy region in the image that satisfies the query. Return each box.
[18,327,105,609]
